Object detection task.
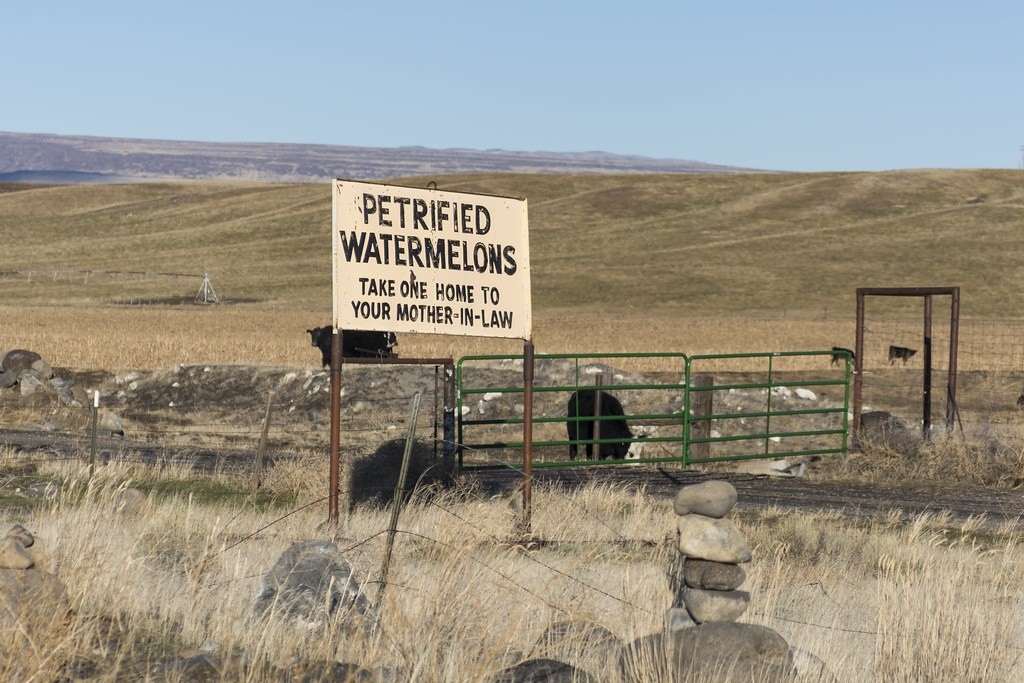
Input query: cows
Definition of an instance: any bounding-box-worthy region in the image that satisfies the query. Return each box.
[566,388,652,467]
[307,325,398,369]
[829,347,856,367]
[889,345,917,366]
